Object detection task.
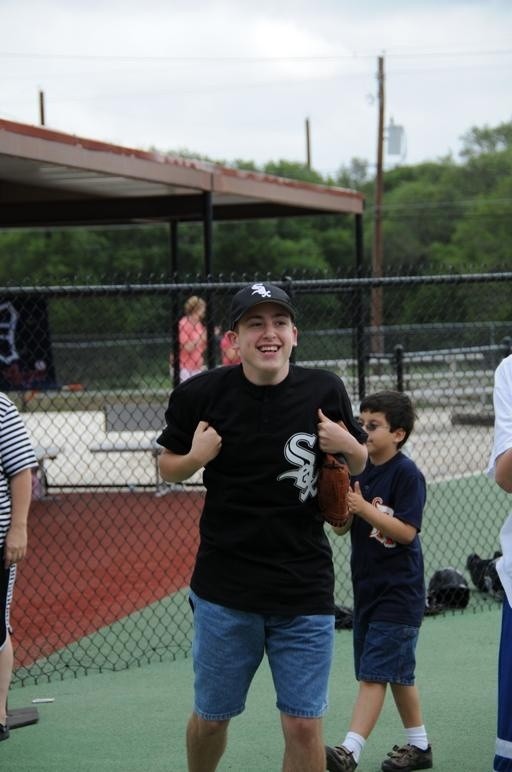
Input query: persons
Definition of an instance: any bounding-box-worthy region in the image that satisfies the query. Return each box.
[0,360,50,391]
[170,294,207,387]
[324,389,433,770]
[157,283,368,772]
[488,353,512,770]
[0,393,39,739]
[220,319,242,366]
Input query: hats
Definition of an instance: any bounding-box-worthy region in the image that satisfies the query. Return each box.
[230,284,295,330]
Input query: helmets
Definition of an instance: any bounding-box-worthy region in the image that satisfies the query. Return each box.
[428,569,469,609]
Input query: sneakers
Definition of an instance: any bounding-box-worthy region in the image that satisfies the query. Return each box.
[325,745,358,772]
[382,744,432,772]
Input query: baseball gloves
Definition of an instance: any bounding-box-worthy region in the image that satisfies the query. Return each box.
[335,605,353,629]
[319,421,351,527]
[424,590,443,615]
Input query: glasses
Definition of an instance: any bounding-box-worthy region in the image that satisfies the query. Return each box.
[358,423,376,431]
